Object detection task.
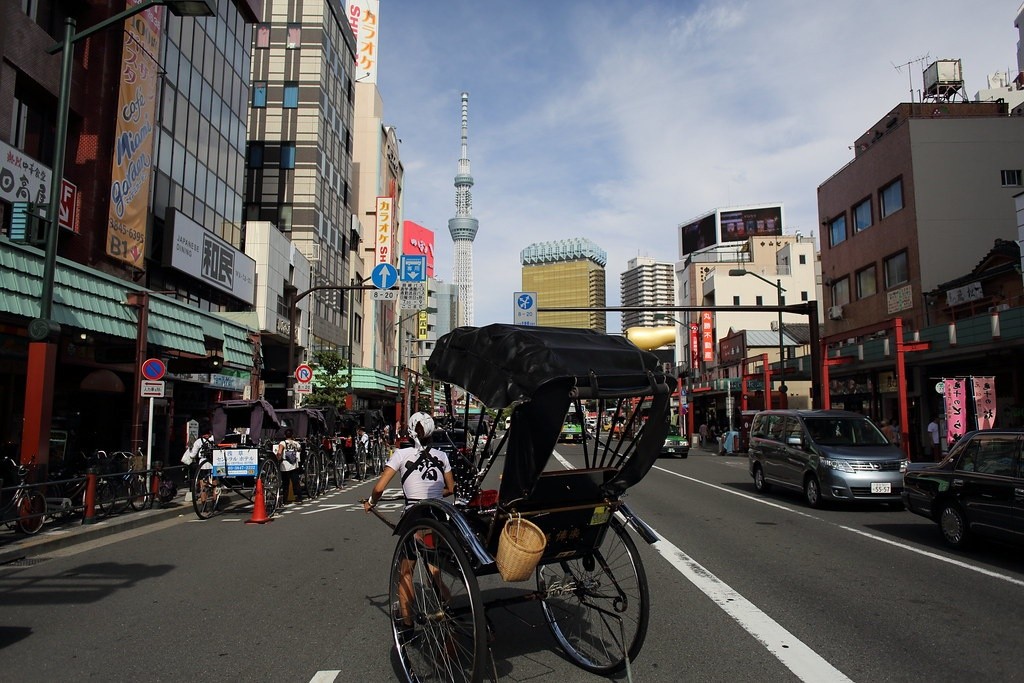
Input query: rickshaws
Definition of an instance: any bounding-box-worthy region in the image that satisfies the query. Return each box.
[192,432,394,520]
[359,323,681,683]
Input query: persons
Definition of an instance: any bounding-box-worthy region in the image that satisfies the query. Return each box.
[698,419,729,449]
[351,425,369,482]
[190,430,214,501]
[277,429,304,504]
[862,416,900,450]
[927,418,939,462]
[363,411,457,632]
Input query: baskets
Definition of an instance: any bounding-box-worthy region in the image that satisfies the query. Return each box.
[497,517,547,583]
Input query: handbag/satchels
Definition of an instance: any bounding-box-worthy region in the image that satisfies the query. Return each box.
[285,451,297,465]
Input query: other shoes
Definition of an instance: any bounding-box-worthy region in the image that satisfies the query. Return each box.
[393,608,415,641]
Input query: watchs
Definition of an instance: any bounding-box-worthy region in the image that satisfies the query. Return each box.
[368,496,376,507]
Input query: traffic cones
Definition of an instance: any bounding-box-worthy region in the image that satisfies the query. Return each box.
[244,478,274,524]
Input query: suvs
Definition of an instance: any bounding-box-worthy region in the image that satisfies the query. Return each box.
[453,419,496,459]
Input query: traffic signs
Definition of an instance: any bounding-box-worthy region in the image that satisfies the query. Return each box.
[295,383,313,393]
[141,380,165,397]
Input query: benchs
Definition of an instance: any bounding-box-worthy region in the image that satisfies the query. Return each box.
[434,467,620,578]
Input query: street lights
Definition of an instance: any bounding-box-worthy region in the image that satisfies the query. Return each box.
[396,307,438,433]
[729,269,788,409]
[14,1,218,536]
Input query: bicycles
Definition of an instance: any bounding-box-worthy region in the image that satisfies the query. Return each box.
[0,454,48,537]
[47,447,149,520]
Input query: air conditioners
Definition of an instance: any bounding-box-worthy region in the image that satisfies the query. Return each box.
[828,306,842,320]
[771,321,778,331]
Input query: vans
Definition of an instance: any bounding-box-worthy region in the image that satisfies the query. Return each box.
[748,409,912,509]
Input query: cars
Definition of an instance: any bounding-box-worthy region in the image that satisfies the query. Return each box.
[634,422,690,459]
[900,427,1024,557]
[430,428,477,466]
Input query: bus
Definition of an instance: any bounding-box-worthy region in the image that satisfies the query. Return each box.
[559,402,586,444]
[504,416,511,430]
